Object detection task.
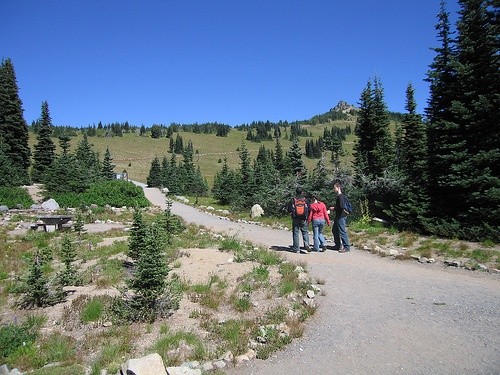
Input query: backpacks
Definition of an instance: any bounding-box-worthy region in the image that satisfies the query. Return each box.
[293,197,306,216]
[341,195,353,214]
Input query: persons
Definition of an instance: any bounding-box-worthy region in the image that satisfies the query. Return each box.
[287,187,312,253]
[307,193,331,251]
[122,171,128,181]
[328,182,350,253]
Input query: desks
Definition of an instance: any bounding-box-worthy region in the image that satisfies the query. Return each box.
[36,215,73,233]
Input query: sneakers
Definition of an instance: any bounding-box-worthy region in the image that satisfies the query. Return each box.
[339,247,350,253]
[331,245,342,250]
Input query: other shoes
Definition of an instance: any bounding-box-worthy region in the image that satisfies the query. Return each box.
[321,246,327,252]
[305,245,312,252]
[293,251,300,253]
[311,248,319,252]
